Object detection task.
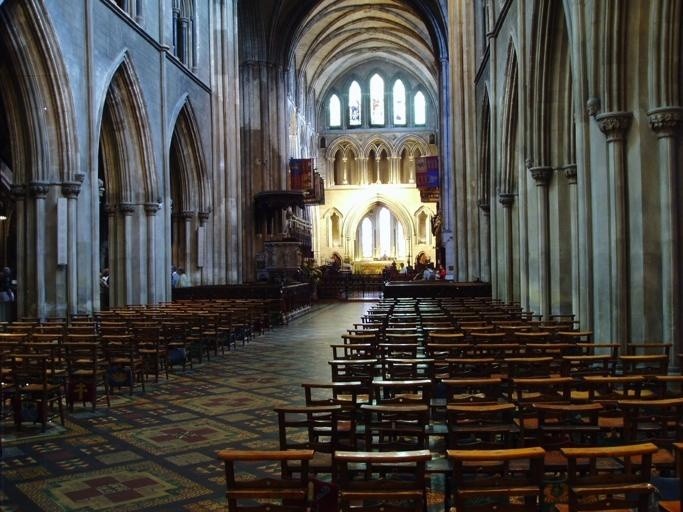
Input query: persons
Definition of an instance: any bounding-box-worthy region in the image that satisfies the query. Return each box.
[0,261,16,305]
[399,260,413,274]
[171,265,190,290]
[333,253,341,270]
[99,268,111,289]
[423,265,446,281]
